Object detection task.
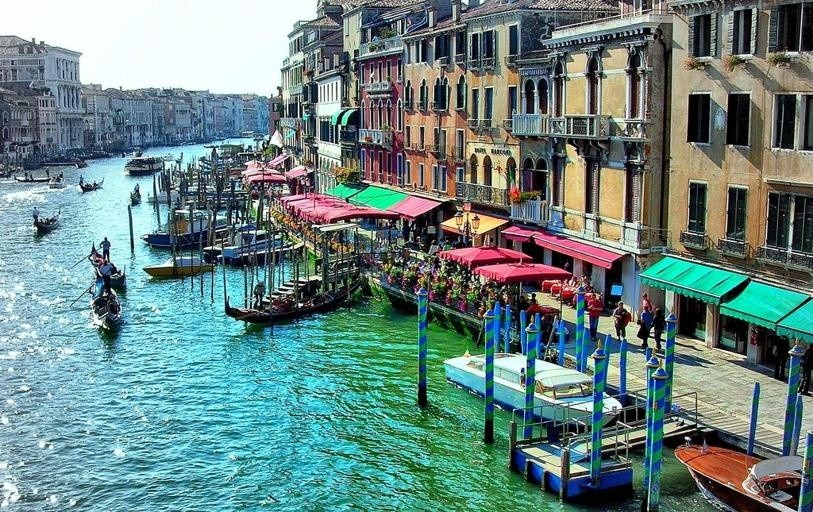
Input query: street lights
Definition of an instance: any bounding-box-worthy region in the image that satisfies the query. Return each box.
[455,209,479,246]
[301,166,311,200]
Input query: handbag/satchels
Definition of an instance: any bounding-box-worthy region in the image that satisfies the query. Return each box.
[637,323,649,338]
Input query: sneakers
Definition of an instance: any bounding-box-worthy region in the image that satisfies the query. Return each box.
[623,339,626,342]
[646,344,648,347]
[656,345,660,348]
[618,339,621,342]
[641,344,645,348]
[592,338,595,341]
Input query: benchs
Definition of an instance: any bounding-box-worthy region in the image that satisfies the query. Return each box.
[742,454,804,505]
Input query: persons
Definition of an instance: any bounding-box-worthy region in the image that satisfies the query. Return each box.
[496,293,537,330]
[99,237,110,262]
[586,294,603,341]
[612,301,627,341]
[551,272,599,310]
[133,184,139,193]
[652,308,665,350]
[772,335,789,381]
[635,306,653,347]
[32,206,38,220]
[24,166,100,192]
[796,342,812,395]
[641,293,652,315]
[99,260,113,289]
[330,219,467,266]
[252,282,265,309]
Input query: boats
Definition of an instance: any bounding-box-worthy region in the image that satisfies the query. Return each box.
[670,436,809,512]
[141,256,216,278]
[77,175,105,192]
[90,278,123,328]
[89,241,127,288]
[224,277,361,325]
[12,171,68,188]
[140,133,364,268]
[441,348,622,432]
[131,192,141,207]
[33,205,62,232]
[124,155,166,177]
